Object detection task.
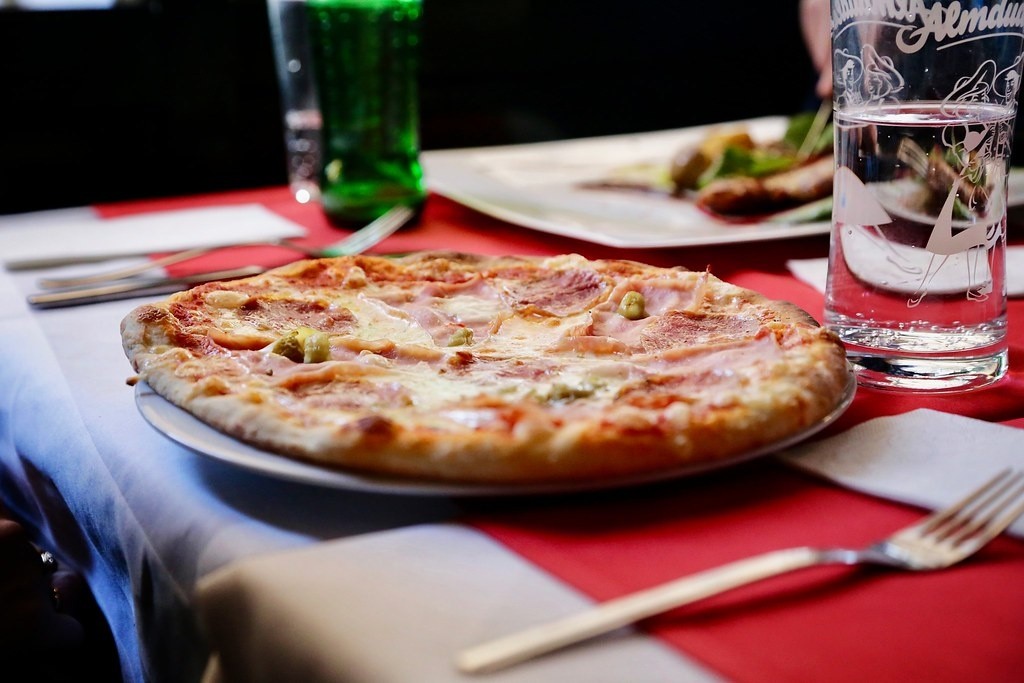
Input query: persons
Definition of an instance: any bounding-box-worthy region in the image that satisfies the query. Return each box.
[800,0,1024,160]
[0,518,82,643]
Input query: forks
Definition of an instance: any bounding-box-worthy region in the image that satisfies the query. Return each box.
[24,203,414,311]
[448,462,1024,678]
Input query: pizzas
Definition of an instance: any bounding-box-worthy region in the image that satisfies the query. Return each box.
[116,249,849,481]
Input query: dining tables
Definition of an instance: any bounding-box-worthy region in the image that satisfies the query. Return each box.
[0,105,1024,683]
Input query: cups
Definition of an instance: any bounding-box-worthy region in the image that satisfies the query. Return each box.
[822,0,1022,396]
[266,0,428,230]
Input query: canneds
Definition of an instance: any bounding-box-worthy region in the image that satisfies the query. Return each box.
[265,0,427,225]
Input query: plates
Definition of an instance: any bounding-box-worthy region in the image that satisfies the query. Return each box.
[413,117,1024,246]
[133,338,857,494]
